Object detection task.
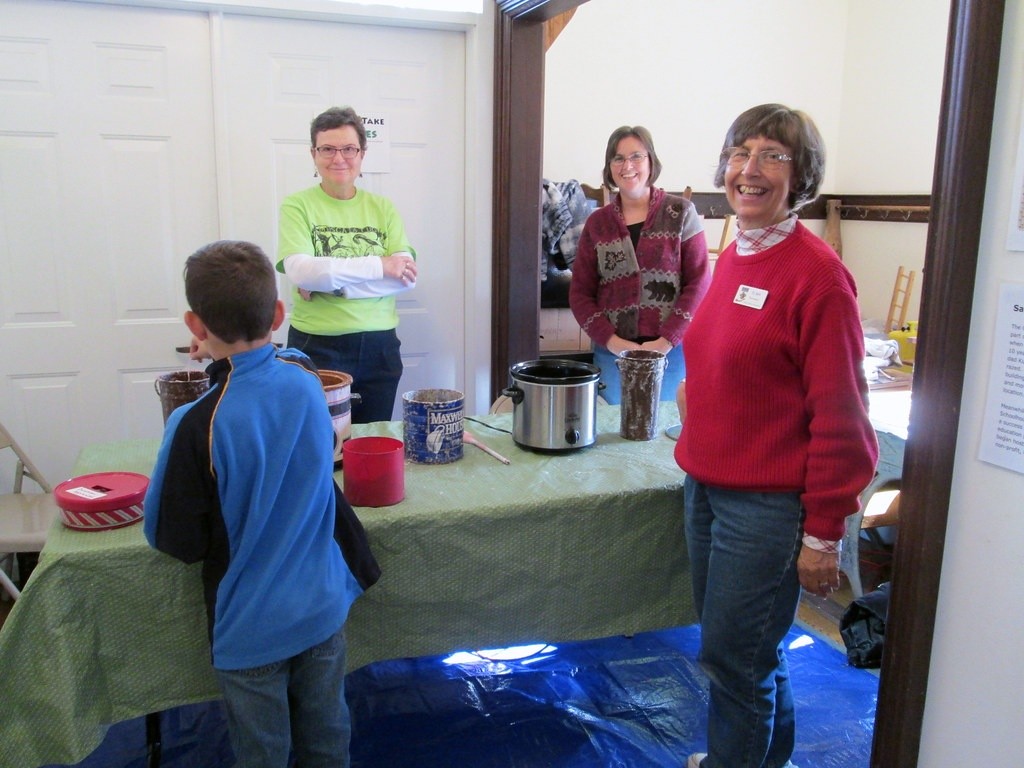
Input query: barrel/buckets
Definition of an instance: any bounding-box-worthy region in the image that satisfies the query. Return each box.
[154,369,211,428]
[614,349,669,441]
[316,370,353,474]
[341,437,405,507]
[402,388,466,465]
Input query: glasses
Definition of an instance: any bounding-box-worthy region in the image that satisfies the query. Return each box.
[721,144,795,169]
[314,146,361,159]
[609,152,650,166]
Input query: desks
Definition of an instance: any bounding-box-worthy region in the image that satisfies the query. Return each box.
[0,398,703,768]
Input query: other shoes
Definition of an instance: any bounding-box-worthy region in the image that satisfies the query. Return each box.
[688,753,710,768]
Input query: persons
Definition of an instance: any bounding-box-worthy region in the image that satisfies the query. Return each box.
[672,103,879,768]
[275,106,418,424]
[142,239,383,767]
[566,125,714,407]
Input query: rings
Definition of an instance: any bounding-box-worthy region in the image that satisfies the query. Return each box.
[404,269,408,273]
[821,582,828,586]
[407,263,409,266]
[402,276,406,279]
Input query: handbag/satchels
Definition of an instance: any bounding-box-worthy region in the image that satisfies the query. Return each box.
[839,581,891,669]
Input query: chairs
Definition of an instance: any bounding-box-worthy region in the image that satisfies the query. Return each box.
[0,420,58,602]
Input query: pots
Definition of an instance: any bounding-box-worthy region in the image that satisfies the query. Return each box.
[502,359,602,455]
